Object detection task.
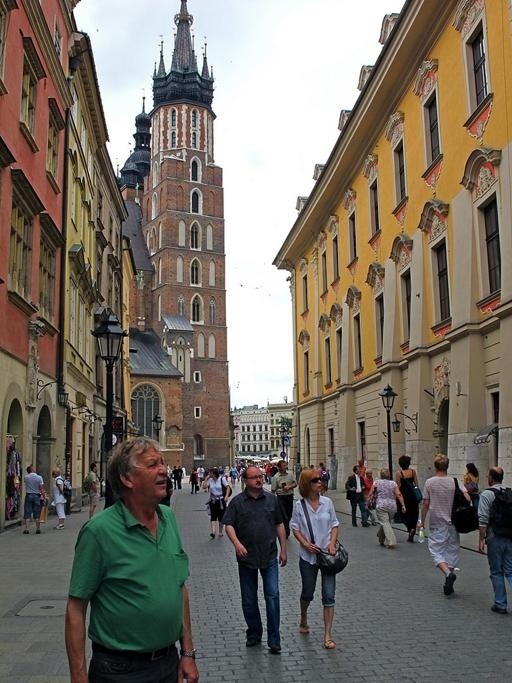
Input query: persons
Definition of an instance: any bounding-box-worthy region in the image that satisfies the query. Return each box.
[415,451,472,598]
[344,464,376,527]
[224,465,288,652]
[369,468,406,545]
[25,463,44,534]
[395,455,419,542]
[189,465,244,538]
[52,468,67,529]
[288,467,340,650]
[257,460,297,536]
[311,463,326,496]
[61,434,202,683]
[464,462,479,501]
[476,465,512,615]
[86,463,100,519]
[160,465,183,506]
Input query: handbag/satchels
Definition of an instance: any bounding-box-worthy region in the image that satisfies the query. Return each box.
[464,481,479,494]
[196,486,199,491]
[366,495,376,510]
[413,485,423,501]
[316,540,348,576]
[38,506,48,523]
[451,488,479,534]
[222,486,232,497]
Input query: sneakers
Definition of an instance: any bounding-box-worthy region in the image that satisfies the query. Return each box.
[36,530,40,534]
[443,573,456,595]
[352,520,357,527]
[210,533,215,538]
[23,530,29,534]
[410,529,415,536]
[361,522,369,527]
[491,605,508,614]
[218,534,224,537]
[53,523,64,530]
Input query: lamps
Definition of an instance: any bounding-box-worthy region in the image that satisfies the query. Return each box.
[36,375,69,406]
[392,412,418,434]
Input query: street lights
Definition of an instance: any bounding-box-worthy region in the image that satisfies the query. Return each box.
[378,382,397,474]
[91,311,127,450]
[150,412,164,440]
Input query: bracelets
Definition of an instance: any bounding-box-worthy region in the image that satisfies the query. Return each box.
[178,646,196,658]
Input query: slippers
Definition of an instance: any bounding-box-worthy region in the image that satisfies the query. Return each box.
[324,638,336,649]
[298,617,309,633]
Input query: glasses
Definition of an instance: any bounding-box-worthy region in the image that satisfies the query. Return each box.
[310,477,322,483]
[247,475,264,480]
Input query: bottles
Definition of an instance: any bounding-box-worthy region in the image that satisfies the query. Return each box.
[418,526,425,544]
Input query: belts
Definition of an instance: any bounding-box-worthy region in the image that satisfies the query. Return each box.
[92,640,176,661]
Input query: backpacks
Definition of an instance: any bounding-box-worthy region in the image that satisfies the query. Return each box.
[56,478,72,500]
[484,487,512,540]
[82,476,92,493]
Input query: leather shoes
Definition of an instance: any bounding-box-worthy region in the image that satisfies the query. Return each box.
[270,645,281,651]
[246,638,257,647]
